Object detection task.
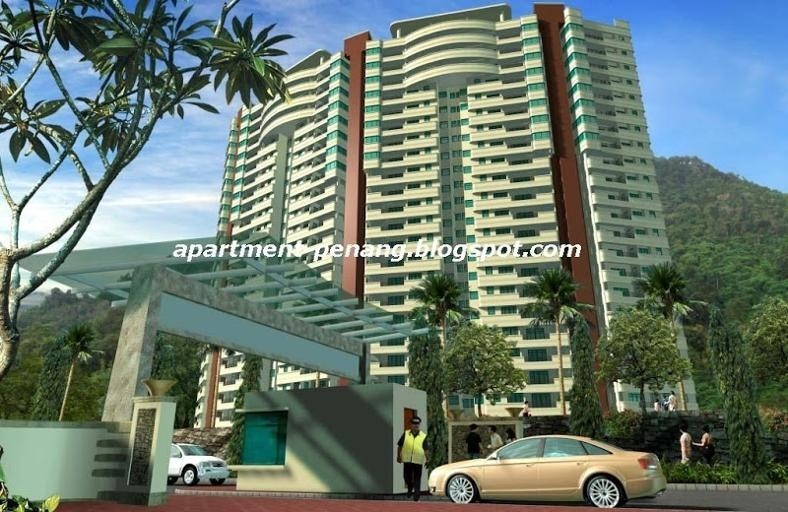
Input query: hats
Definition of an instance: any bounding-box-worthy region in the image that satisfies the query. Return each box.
[411,416,421,424]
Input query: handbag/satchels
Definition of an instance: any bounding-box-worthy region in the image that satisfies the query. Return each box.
[701,444,715,456]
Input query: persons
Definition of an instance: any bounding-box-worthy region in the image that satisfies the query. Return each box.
[505,427,517,444]
[654,391,678,411]
[396,415,429,502]
[679,423,693,464]
[486,425,503,451]
[465,423,484,459]
[518,400,532,417]
[692,425,713,465]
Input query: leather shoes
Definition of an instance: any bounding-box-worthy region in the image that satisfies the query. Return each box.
[407,488,420,501]
